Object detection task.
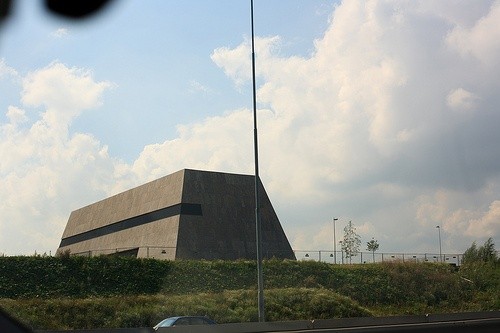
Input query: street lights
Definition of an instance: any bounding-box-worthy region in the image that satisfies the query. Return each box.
[334,218,339,264]
[436,226,445,264]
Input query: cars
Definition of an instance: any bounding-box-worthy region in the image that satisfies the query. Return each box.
[154,315,218,332]
